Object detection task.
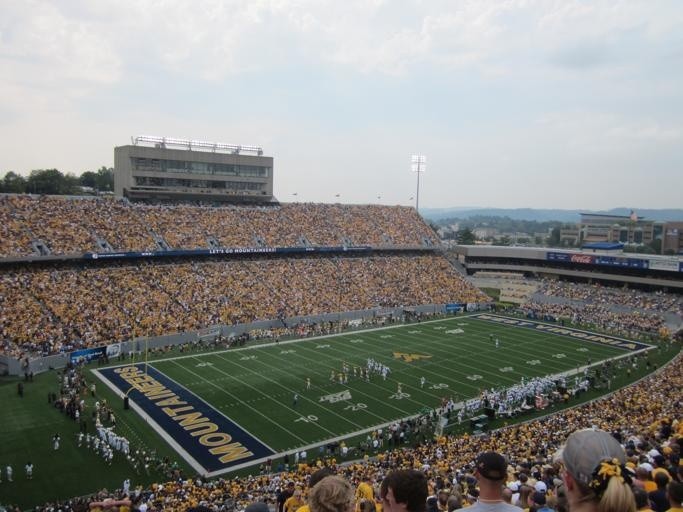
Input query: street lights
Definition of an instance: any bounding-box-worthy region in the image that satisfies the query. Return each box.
[412,154,425,215]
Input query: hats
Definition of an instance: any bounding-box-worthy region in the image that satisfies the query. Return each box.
[454,432,673,504]
[552,428,625,485]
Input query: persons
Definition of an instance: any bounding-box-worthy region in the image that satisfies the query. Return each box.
[0,193,682,512]
